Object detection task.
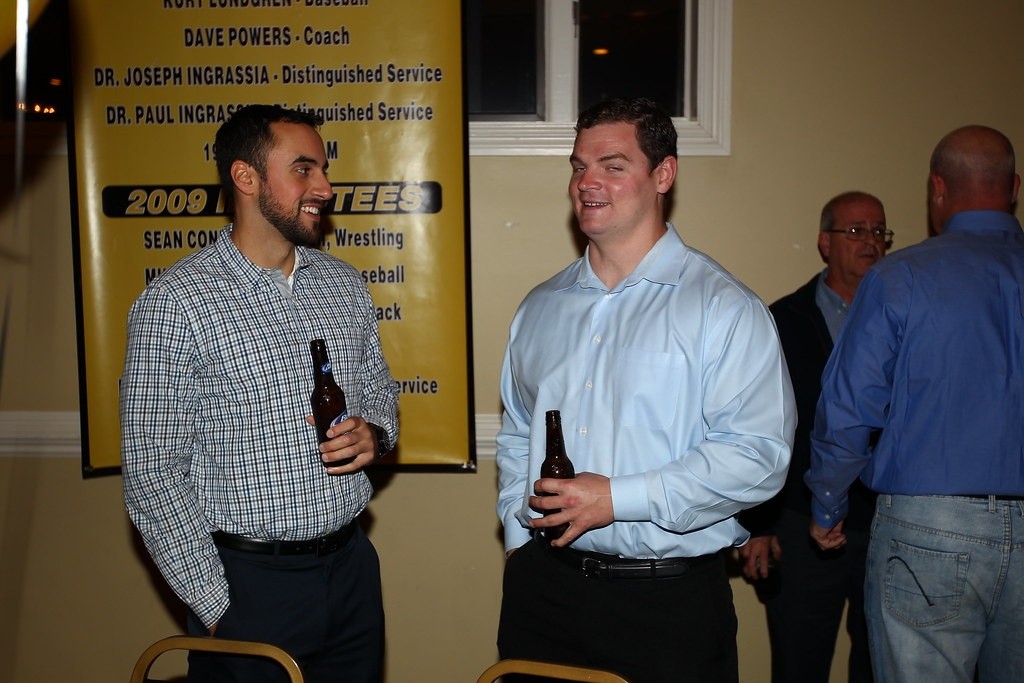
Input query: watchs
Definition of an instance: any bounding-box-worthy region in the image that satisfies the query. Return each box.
[368,422,391,465]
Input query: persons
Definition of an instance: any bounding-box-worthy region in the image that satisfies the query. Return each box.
[733,190,895,683]
[802,125,1023,682]
[116,104,402,683]
[494,88,801,683]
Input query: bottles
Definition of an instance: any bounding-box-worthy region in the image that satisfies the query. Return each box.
[309,340,353,468]
[541,409,576,542]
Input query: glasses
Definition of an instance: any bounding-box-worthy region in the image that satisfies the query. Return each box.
[824,225,893,243]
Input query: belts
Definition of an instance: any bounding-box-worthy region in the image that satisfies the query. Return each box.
[532,529,699,581]
[212,516,361,556]
[954,494,1022,502]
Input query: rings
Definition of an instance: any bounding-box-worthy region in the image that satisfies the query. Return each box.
[820,546,826,551]
